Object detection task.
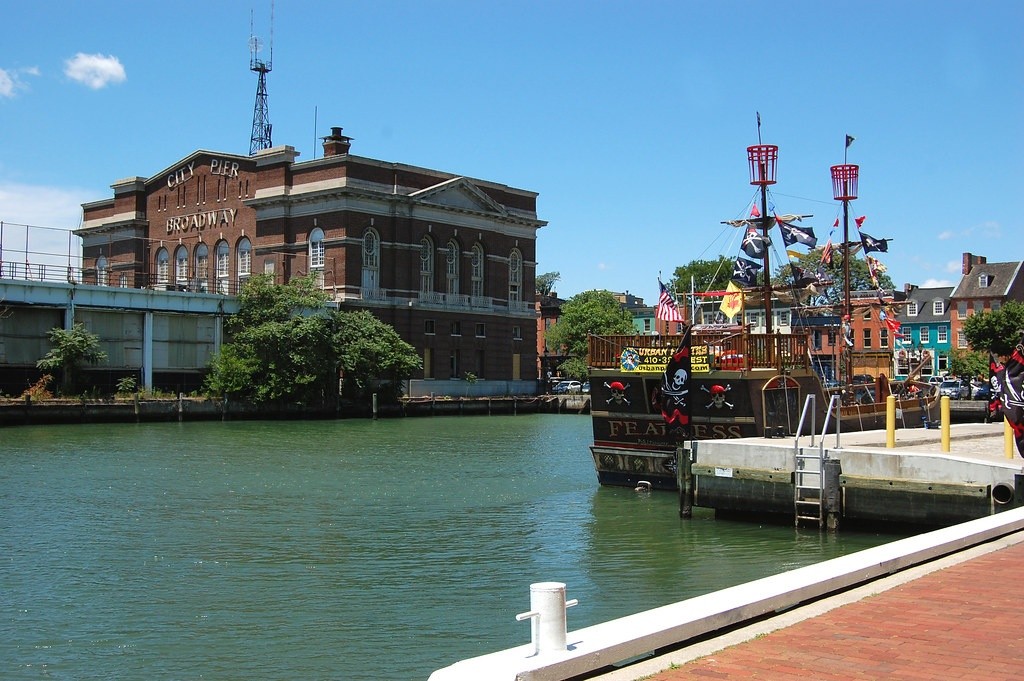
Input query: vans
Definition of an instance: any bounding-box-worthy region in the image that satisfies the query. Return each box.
[551,380,581,395]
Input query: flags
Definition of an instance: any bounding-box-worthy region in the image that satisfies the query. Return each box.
[989,352,1005,420]
[768,201,775,212]
[775,215,818,250]
[732,257,763,287]
[787,250,806,261]
[790,263,819,288]
[750,204,761,218]
[855,216,866,228]
[846,134,855,147]
[867,256,905,338]
[657,280,685,323]
[658,323,691,425]
[741,226,771,259]
[860,232,888,254]
[757,112,761,128]
[720,281,747,319]
[820,218,840,265]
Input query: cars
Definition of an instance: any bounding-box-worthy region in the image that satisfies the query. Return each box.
[917,374,990,401]
[581,382,590,394]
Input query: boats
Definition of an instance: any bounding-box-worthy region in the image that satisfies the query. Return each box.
[584,108,945,495]
